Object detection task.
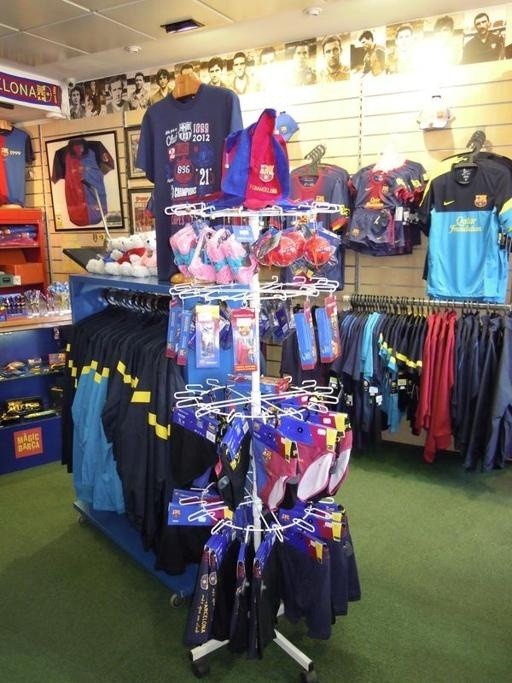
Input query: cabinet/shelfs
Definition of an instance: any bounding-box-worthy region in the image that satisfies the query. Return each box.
[0,310,72,474]
[0,208,48,305]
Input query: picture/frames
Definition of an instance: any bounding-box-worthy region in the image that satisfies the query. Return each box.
[44,131,125,231]
[124,124,148,180]
[127,188,156,235]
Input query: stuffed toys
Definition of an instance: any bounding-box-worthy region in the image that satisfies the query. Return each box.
[85,230,159,276]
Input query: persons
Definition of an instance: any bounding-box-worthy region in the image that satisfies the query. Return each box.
[68,11,506,118]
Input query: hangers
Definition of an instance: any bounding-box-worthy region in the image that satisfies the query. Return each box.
[349,294,508,324]
[104,287,172,327]
[299,145,326,176]
[455,131,486,167]
